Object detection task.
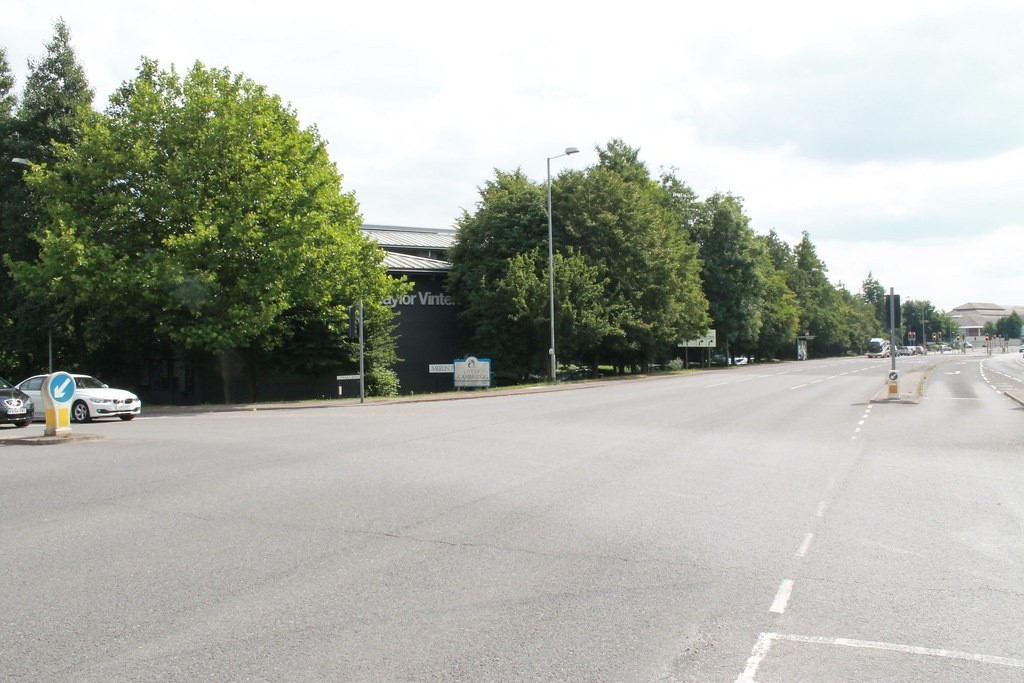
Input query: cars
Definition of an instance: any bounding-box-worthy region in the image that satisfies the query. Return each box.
[14,373,142,424]
[893,342,972,358]
[0,377,34,427]
[1019,345,1024,352]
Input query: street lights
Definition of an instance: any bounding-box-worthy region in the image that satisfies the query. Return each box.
[547,145,581,381]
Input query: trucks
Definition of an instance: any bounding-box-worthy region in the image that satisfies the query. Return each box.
[869,338,890,358]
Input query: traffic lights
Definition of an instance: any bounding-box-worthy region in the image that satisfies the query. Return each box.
[957,334,960,340]
[986,334,989,340]
[933,333,936,341]
